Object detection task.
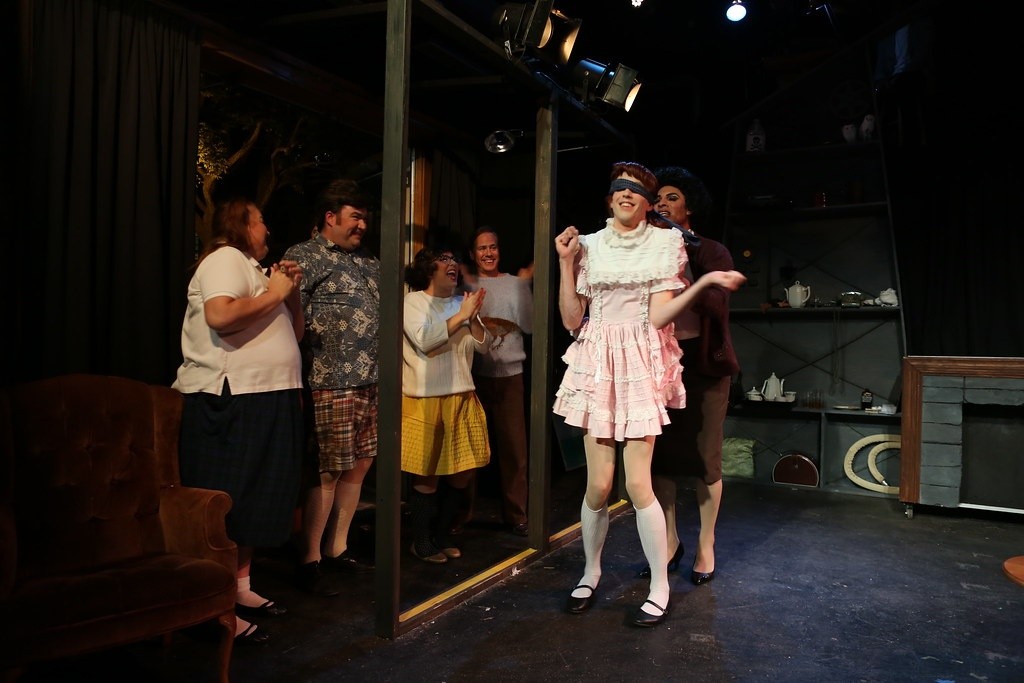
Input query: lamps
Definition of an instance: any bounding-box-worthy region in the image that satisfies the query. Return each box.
[492,0,582,74]
[572,58,643,112]
[485,130,536,153]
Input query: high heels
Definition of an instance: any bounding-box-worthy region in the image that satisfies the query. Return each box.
[690,568,715,585]
[639,540,684,577]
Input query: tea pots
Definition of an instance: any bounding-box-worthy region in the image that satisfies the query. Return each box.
[761,372,785,401]
[784,281,810,308]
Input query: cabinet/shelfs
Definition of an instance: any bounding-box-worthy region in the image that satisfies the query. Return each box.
[719,89,906,500]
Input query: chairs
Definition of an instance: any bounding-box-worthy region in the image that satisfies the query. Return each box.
[0,375,237,683]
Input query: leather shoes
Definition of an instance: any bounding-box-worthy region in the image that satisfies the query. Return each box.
[324,550,375,574]
[299,562,339,595]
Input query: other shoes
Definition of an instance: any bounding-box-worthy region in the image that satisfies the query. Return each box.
[447,516,464,533]
[633,600,671,626]
[411,542,448,564]
[234,623,270,643]
[236,596,289,615]
[567,580,600,614]
[444,547,460,558]
[507,521,529,535]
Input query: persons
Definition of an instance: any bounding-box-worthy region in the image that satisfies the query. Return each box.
[168,198,308,645]
[452,232,531,537]
[399,245,493,565]
[636,164,742,587]
[276,178,381,601]
[554,161,748,624]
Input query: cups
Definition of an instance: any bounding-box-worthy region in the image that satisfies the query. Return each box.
[784,392,796,402]
[747,386,760,401]
[813,191,831,208]
[802,390,824,409]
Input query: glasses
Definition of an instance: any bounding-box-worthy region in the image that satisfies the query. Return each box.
[434,255,462,264]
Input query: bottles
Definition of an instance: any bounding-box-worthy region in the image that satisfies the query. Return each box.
[745,118,766,152]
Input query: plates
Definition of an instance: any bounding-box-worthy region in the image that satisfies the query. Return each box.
[834,406,861,410]
